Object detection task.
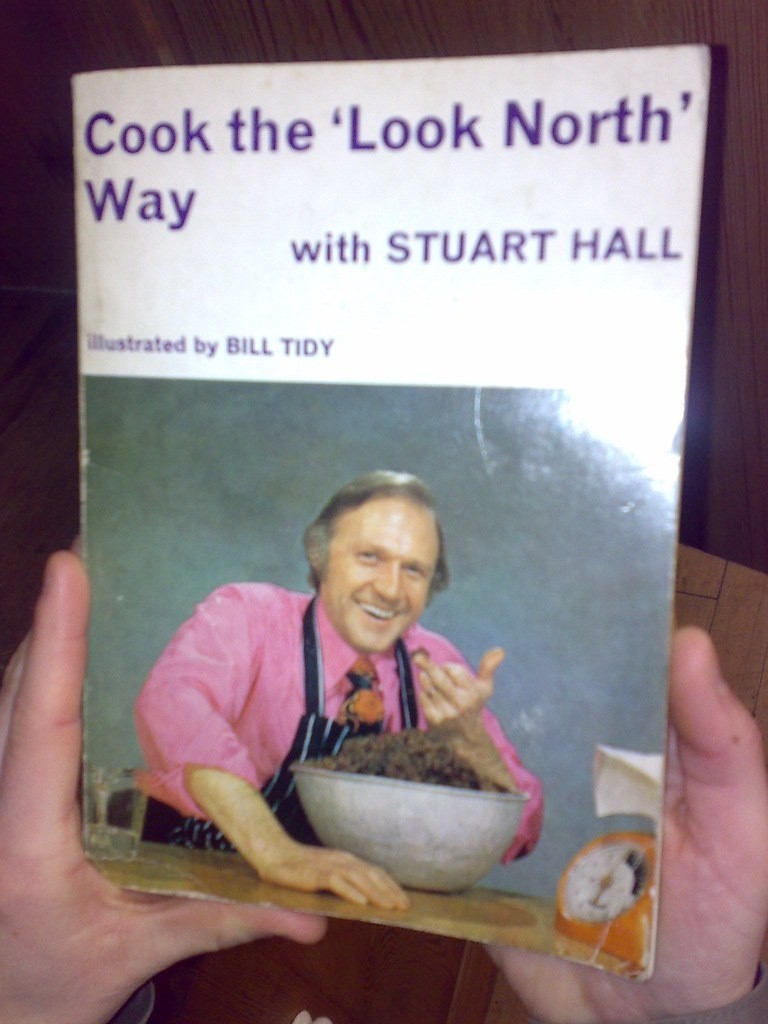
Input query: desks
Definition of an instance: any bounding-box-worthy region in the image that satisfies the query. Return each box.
[87,842,560,954]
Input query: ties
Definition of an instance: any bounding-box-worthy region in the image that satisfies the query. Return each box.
[336,655,388,733]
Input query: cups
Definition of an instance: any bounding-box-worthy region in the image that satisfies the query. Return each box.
[83,758,151,863]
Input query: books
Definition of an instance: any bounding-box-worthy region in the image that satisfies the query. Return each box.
[73,42,710,983]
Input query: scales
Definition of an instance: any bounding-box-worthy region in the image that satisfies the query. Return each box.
[553,743,665,968]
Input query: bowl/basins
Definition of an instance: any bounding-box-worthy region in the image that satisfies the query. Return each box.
[289,765,532,892]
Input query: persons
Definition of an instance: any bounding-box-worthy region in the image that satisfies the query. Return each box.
[0,532,768,1024]
[133,469,545,912]
